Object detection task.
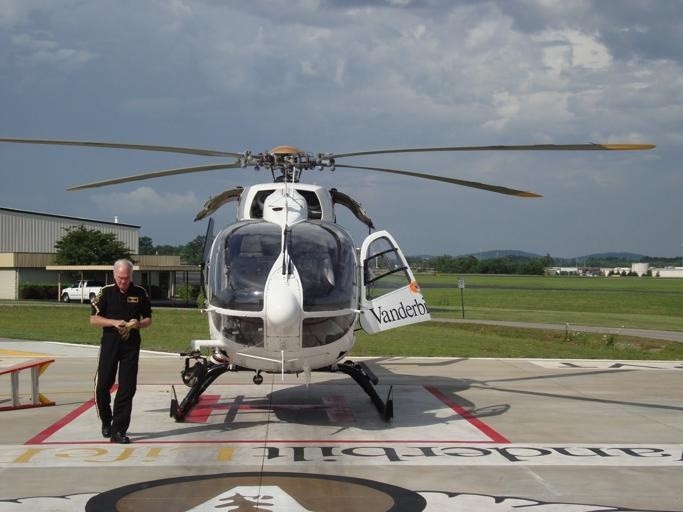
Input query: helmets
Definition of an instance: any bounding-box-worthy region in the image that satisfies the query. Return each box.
[182,364,205,387]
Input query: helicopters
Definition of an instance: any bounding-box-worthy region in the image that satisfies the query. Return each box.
[0,138,659,425]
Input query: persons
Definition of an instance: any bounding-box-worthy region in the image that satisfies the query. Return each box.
[87,259,152,444]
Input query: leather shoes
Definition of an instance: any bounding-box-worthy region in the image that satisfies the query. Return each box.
[111,432,129,443]
[102,417,111,437]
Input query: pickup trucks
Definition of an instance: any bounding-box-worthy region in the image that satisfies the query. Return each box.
[59,279,106,303]
